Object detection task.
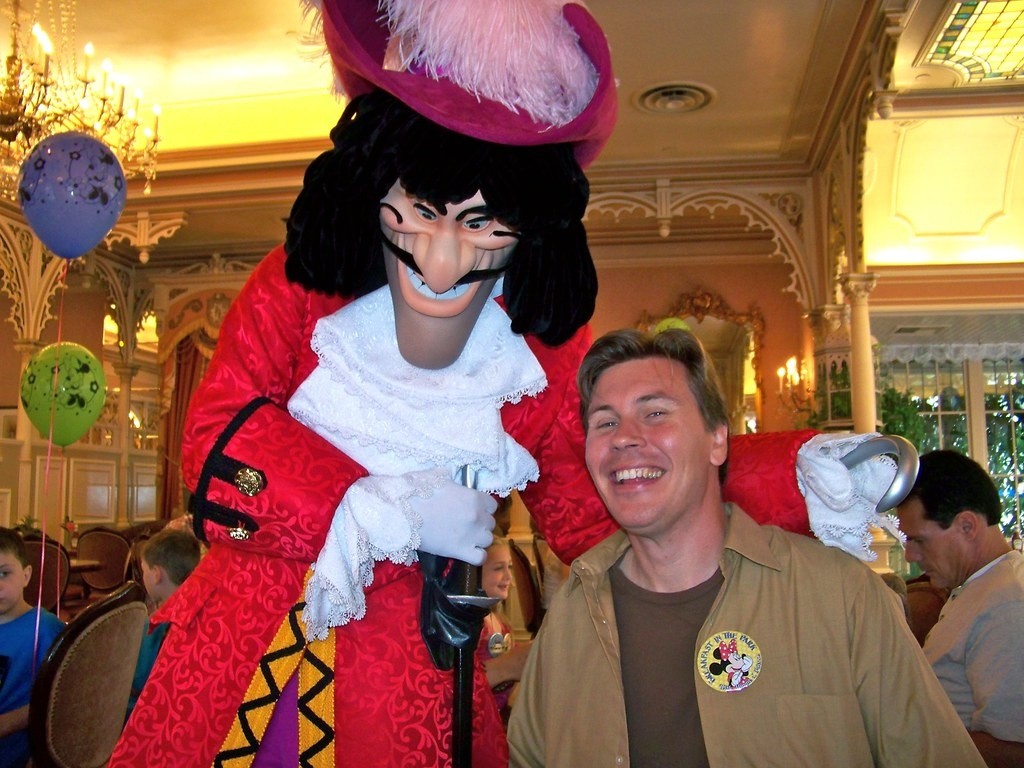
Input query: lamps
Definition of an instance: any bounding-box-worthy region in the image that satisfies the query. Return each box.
[778,356,811,413]
[0,0,165,201]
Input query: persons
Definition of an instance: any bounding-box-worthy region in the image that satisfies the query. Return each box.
[896,450,1024,768]
[127,529,202,712]
[506,328,1024,768]
[0,526,65,768]
[109,0,919,768]
[475,535,535,768]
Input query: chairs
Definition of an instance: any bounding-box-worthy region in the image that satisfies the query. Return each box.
[14,521,161,768]
[508,531,564,633]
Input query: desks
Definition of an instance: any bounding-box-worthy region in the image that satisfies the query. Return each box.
[68,557,101,576]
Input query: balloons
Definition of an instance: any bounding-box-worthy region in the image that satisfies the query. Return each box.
[19,341,106,449]
[19,134,126,260]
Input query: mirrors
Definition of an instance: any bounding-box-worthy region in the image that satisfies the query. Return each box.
[637,292,765,440]
